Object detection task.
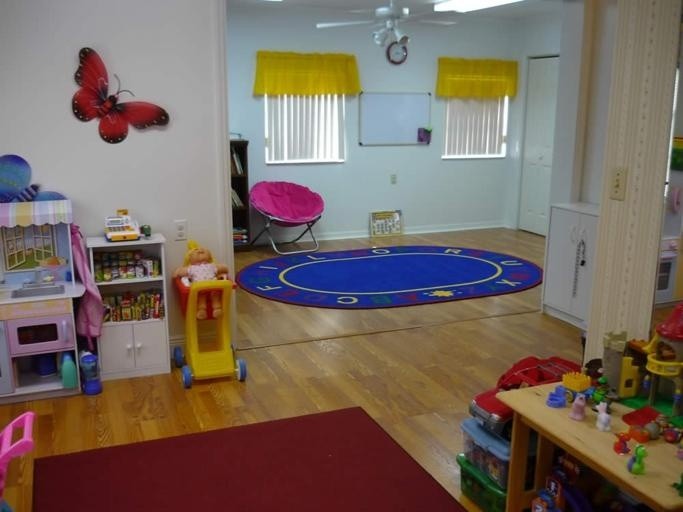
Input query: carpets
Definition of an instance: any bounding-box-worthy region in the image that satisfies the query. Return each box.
[30,405,470,512]
[236,244,543,311]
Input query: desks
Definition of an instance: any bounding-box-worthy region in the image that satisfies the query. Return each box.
[491,374,682,511]
[1,282,85,403]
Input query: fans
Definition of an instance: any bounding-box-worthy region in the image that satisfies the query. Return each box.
[314,0,458,37]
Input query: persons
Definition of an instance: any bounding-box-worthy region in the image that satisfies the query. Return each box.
[173,246,229,320]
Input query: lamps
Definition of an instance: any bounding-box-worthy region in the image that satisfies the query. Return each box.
[374,0,412,46]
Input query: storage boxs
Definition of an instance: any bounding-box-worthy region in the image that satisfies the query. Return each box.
[452,387,564,512]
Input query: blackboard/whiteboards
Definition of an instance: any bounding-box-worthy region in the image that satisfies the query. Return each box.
[359,91,432,146]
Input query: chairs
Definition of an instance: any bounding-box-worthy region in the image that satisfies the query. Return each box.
[249,179,324,255]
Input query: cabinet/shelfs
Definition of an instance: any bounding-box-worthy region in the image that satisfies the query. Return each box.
[544,200,600,335]
[230,138,250,249]
[84,233,172,381]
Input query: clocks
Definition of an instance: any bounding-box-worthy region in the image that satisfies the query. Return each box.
[386,40,407,66]
